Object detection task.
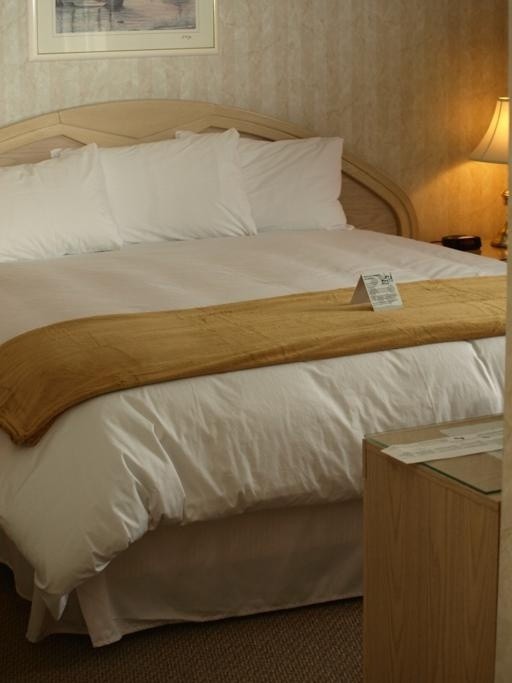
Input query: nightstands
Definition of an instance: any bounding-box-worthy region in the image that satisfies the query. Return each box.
[435,238,508,263]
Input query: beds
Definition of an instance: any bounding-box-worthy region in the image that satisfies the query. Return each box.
[0,98,506,649]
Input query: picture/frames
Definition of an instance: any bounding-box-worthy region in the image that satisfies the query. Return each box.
[28,0,220,62]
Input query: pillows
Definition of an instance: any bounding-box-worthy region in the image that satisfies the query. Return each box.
[49,126,257,244]
[174,131,354,230]
[0,140,123,265]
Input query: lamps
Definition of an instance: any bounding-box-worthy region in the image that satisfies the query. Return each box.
[467,97,511,247]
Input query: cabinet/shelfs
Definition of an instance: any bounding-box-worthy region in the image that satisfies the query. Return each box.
[363,413,505,682]
